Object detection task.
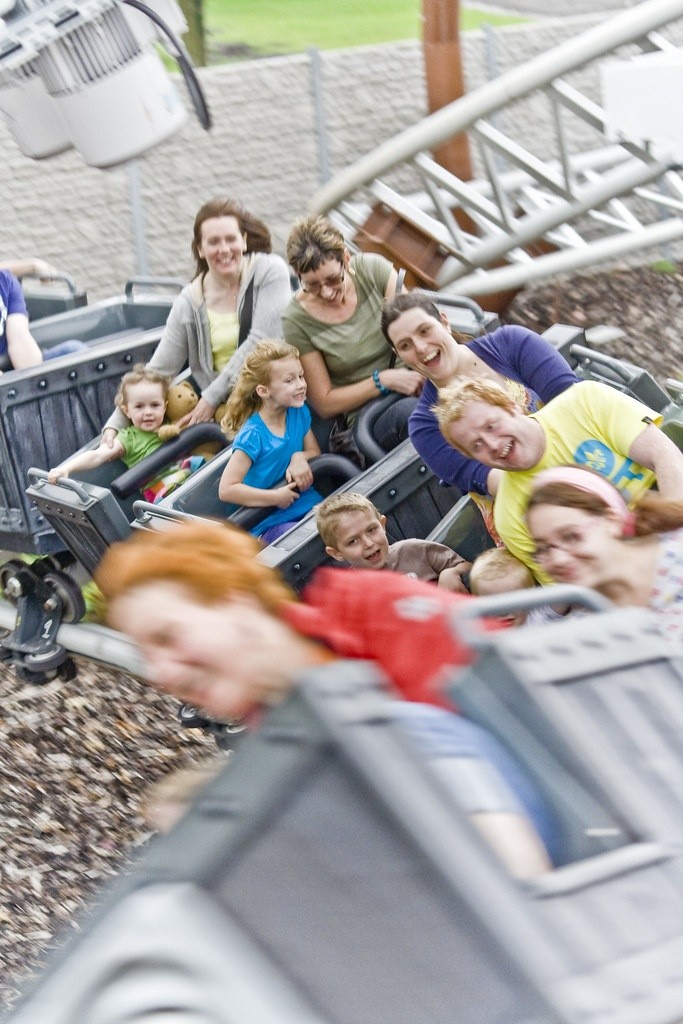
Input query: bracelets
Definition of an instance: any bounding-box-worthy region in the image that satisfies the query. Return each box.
[373,368,388,394]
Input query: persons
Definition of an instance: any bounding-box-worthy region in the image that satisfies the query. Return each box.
[217,340,324,545]
[0,258,90,375]
[316,493,472,599]
[432,376,683,630]
[100,197,294,462]
[380,292,578,547]
[99,521,573,867]
[281,213,420,452]
[48,363,205,504]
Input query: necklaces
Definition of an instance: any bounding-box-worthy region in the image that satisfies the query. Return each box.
[228,291,237,300]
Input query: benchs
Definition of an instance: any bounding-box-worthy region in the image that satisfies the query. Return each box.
[259,324,683,605]
[0,583,683,1024]
[27,361,229,583]
[0,278,185,535]
[130,283,499,541]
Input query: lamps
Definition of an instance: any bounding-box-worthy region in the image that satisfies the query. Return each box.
[0,0,214,170]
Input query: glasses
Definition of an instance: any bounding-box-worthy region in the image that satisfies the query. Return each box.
[298,259,347,295]
[532,515,598,566]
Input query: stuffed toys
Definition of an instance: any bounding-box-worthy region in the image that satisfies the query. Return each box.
[158,380,228,463]
